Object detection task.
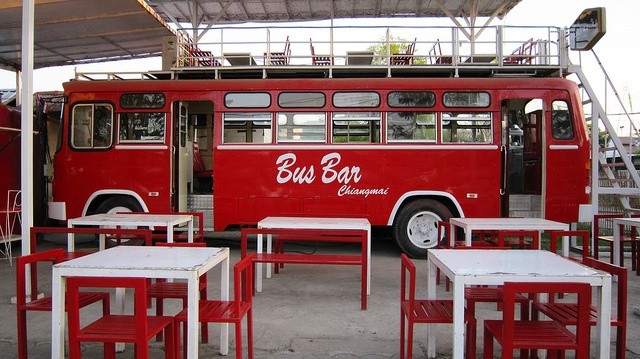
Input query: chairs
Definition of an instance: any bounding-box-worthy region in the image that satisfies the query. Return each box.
[549,230,589,303]
[264,36,291,66]
[490,231,538,249]
[174,255,253,359]
[531,257,627,359]
[429,39,461,64]
[437,221,455,291]
[310,38,334,66]
[134,243,208,343]
[193,142,213,194]
[188,33,221,66]
[484,282,590,359]
[497,230,539,311]
[454,246,529,359]
[400,254,476,359]
[631,214,640,271]
[17,249,109,359]
[67,276,174,359]
[454,225,492,246]
[503,38,533,65]
[594,214,640,276]
[390,38,416,65]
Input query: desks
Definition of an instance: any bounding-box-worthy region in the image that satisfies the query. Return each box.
[449,218,569,287]
[427,249,612,359]
[464,54,496,63]
[257,217,371,295]
[68,214,193,282]
[52,246,229,359]
[222,52,257,65]
[613,218,640,282]
[345,51,374,66]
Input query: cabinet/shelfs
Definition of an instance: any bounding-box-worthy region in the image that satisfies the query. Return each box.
[0,190,22,267]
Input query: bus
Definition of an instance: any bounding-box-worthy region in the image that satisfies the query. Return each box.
[35,70,592,260]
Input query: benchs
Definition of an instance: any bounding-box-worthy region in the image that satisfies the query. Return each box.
[105,212,203,249]
[241,228,367,310]
[275,235,362,274]
[30,227,152,308]
